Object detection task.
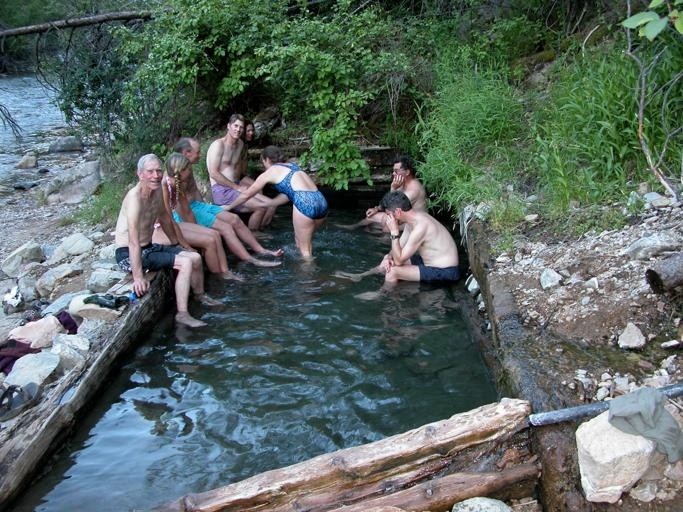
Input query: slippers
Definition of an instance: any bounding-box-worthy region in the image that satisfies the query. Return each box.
[84,294,130,309]
[0,382,43,422]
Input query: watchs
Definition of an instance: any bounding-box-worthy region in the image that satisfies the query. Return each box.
[390,234,399,239]
[374,205,379,212]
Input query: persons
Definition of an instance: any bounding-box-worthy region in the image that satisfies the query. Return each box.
[152,154,245,281]
[115,154,222,328]
[206,114,275,229]
[222,145,329,260]
[336,191,459,300]
[240,120,263,195]
[164,137,284,268]
[333,157,427,233]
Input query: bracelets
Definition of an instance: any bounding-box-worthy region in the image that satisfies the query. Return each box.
[170,243,179,247]
